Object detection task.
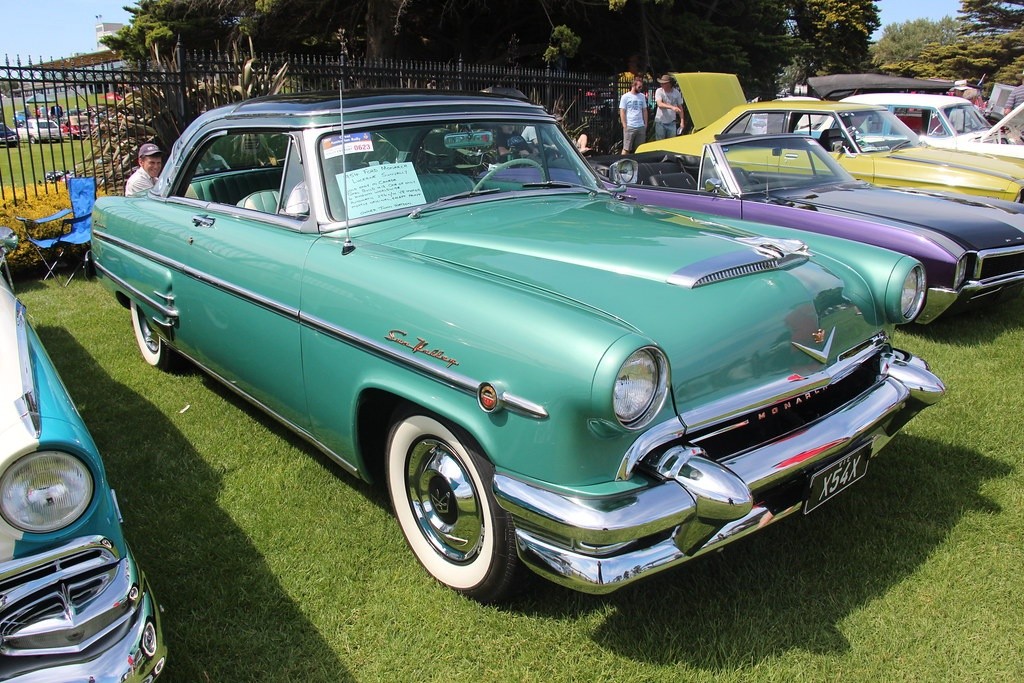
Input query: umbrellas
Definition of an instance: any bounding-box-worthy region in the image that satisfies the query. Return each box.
[101,92,123,100]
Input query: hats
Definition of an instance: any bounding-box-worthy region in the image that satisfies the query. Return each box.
[139,144,163,158]
[657,75,671,83]
[949,80,973,91]
[1016,71,1024,79]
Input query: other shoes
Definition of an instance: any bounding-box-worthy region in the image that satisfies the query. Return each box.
[579,148,592,156]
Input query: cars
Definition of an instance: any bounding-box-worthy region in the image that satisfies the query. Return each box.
[0,122,18,148]
[631,70,1024,209]
[12,119,66,145]
[61,106,106,141]
[0,273,171,683]
[84,92,948,606]
[792,90,1024,175]
[583,98,621,116]
[476,133,1024,329]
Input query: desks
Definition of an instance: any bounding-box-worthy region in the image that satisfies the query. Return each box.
[0,227,22,292]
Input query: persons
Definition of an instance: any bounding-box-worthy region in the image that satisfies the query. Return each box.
[943,90,983,136]
[26,104,33,119]
[36,104,46,118]
[619,79,648,155]
[125,144,161,196]
[1004,71,1024,136]
[496,125,558,162]
[554,113,588,152]
[51,104,63,119]
[655,75,684,140]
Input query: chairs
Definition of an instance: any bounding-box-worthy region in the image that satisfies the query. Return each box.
[649,172,697,190]
[418,172,476,205]
[236,188,279,215]
[15,178,97,287]
[706,166,754,198]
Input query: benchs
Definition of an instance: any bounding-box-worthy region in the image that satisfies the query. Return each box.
[182,166,285,205]
[630,161,688,185]
